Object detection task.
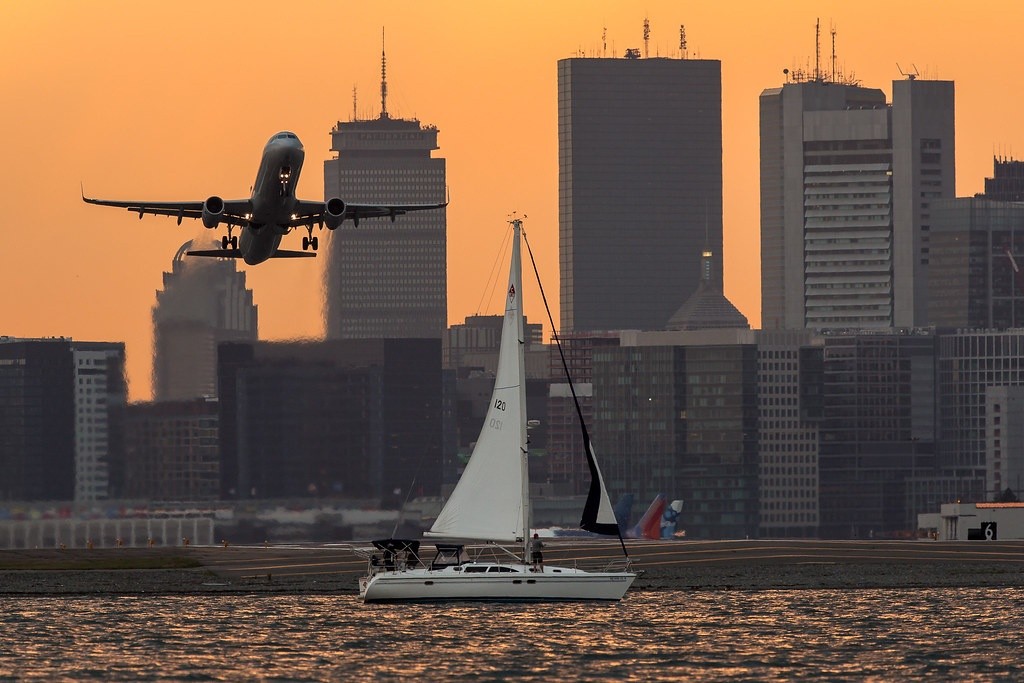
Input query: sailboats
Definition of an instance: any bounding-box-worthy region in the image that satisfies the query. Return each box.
[358,211,645,602]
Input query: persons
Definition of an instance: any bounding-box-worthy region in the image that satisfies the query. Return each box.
[531,533,546,575]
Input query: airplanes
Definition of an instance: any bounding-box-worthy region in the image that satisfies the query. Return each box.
[80,131,451,265]
